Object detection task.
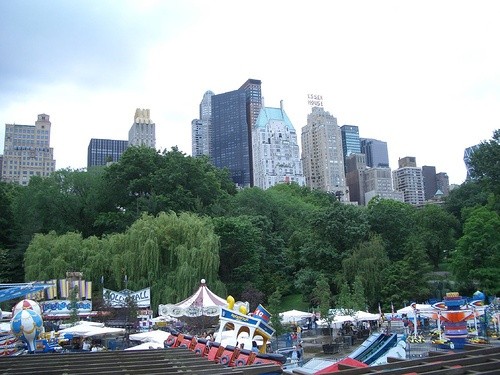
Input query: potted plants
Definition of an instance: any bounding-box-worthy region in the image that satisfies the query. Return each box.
[319,291,361,354]
